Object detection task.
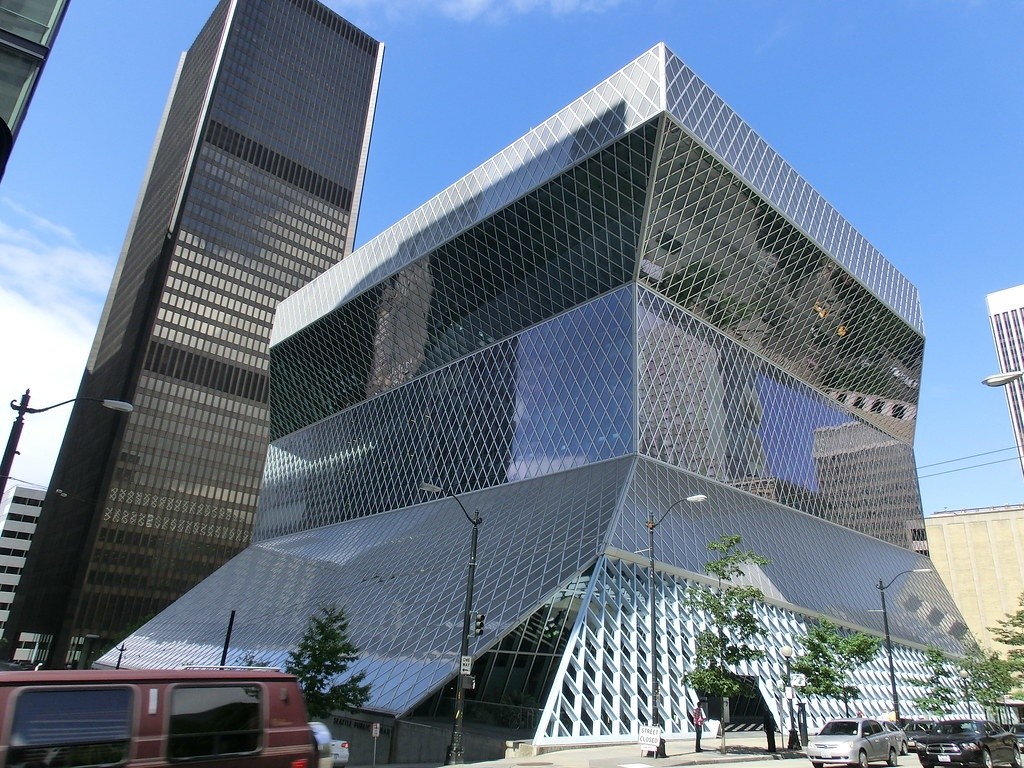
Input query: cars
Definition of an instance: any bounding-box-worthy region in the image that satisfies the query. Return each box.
[876,720,908,757]
[806,717,899,768]
[1009,723,1024,754]
[901,721,939,753]
[916,720,1023,767]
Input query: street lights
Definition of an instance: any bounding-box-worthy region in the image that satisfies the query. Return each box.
[959,669,972,719]
[875,568,934,726]
[0,388,134,502]
[85,634,126,670]
[419,483,484,766]
[782,646,802,750]
[645,495,706,728]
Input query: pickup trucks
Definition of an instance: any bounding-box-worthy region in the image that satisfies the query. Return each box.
[308,721,349,768]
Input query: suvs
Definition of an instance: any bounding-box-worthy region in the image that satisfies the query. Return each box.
[0,663,334,768]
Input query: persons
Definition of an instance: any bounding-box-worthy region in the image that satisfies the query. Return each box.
[693,702,707,753]
[857,711,863,718]
